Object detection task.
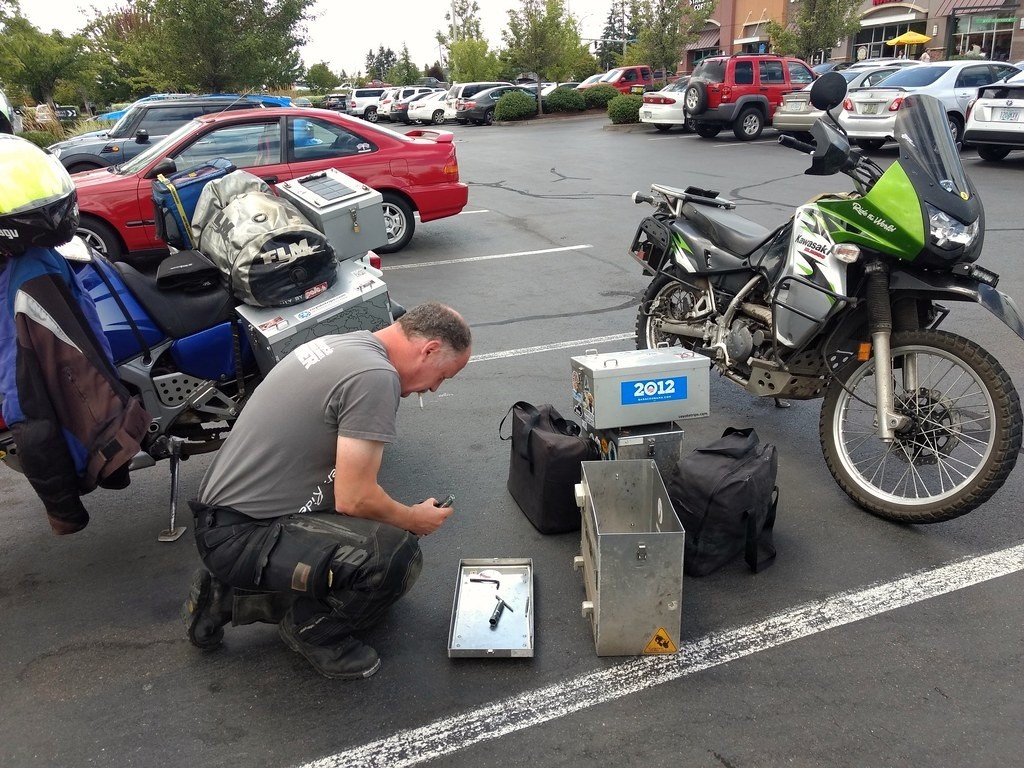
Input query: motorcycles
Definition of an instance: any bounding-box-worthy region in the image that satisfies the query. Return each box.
[34,166,412,545]
[629,71,1024,525]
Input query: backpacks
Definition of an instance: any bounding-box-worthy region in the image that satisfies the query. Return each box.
[190,170,337,307]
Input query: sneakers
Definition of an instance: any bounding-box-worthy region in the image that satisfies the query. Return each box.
[278,602,380,679]
[182,569,225,648]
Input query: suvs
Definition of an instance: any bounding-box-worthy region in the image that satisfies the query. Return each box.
[44,92,325,173]
[682,52,821,141]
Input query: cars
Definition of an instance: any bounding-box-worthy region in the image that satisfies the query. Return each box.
[332,74,584,126]
[774,57,926,134]
[318,94,347,113]
[67,106,470,270]
[964,68,1024,161]
[638,75,696,130]
[292,97,315,108]
[833,59,1021,154]
[976,61,1024,87]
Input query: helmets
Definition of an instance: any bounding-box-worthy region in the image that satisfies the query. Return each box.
[0,133,80,254]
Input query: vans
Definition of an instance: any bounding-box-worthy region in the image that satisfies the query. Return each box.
[35,104,53,124]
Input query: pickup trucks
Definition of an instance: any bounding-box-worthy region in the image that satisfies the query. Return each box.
[576,64,680,96]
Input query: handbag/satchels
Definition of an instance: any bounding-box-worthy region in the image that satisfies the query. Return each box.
[498,400,601,535]
[669,427,780,577]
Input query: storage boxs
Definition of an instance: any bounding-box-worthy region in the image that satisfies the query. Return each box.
[235,258,398,379]
[274,167,387,259]
[572,343,711,657]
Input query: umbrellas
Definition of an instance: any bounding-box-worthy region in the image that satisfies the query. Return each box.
[691,53,714,64]
[886,30,932,59]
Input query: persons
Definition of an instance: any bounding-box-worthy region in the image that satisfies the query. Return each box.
[896,49,906,59]
[185,303,471,680]
[919,48,930,63]
[965,41,986,57]
